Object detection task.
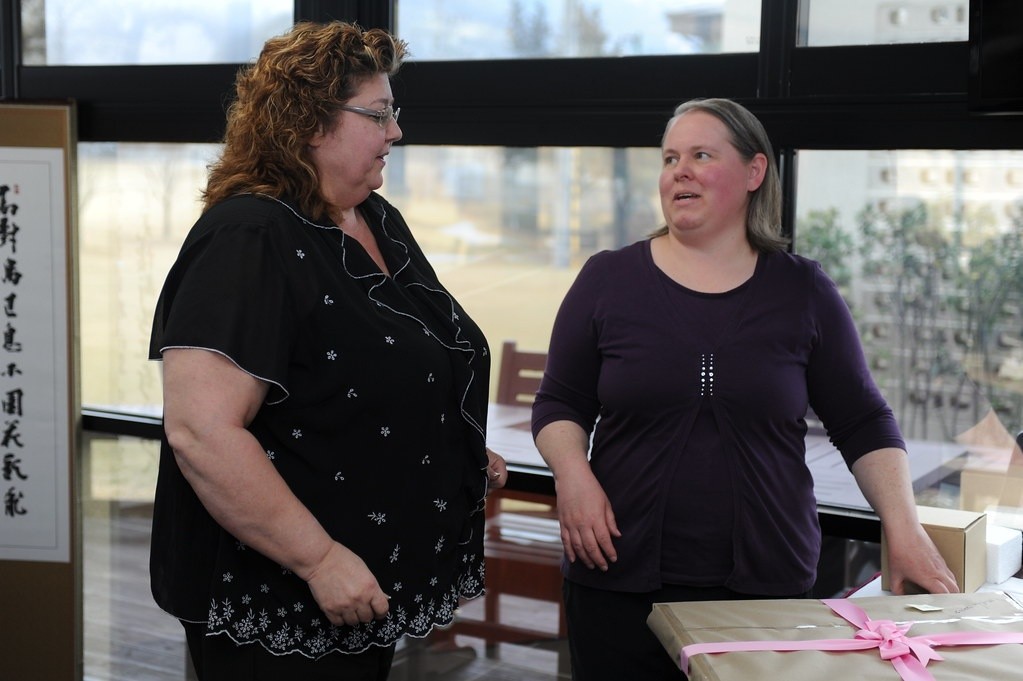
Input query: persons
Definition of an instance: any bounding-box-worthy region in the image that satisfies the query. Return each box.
[531,97,960,681]
[147,22,509,680]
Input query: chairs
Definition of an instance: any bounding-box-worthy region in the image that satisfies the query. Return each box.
[433,341,590,668]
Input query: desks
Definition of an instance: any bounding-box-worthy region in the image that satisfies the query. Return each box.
[75,399,967,594]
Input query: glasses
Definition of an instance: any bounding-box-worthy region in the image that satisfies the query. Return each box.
[327,103,401,128]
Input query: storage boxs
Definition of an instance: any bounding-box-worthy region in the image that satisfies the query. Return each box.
[880,455,1023,596]
